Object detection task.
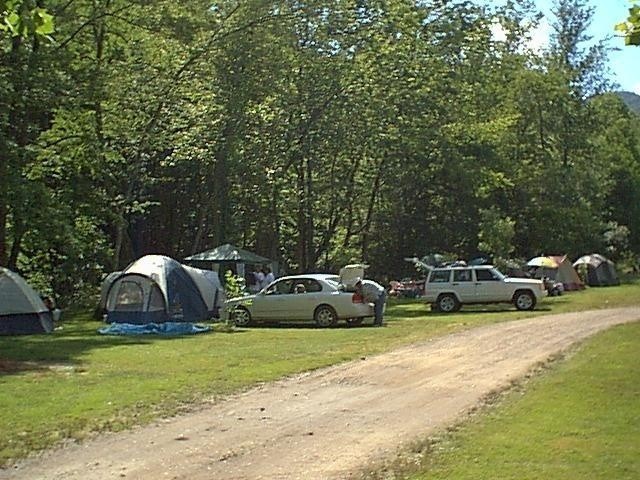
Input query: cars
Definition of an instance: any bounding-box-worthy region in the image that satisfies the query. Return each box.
[224,264,386,328]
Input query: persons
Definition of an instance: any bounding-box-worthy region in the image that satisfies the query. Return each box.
[353,276,386,328]
[247,263,278,293]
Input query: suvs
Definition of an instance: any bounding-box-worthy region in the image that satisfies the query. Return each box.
[425,265,544,312]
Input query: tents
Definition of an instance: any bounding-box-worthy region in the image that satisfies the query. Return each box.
[95,253,227,325]
[394,249,620,298]
[182,243,290,294]
[0,266,56,336]
